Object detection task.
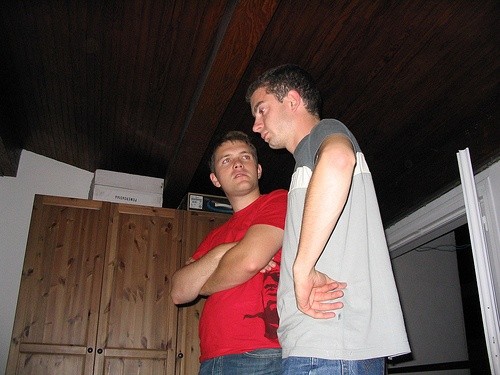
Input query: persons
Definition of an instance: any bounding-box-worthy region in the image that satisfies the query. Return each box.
[170,131,294,374]
[244,66,412,375]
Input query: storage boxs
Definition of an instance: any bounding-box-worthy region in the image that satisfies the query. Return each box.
[88,168,165,208]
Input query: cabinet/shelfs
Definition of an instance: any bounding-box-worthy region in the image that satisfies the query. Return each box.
[4,194,184,375]
[179,209,232,374]
